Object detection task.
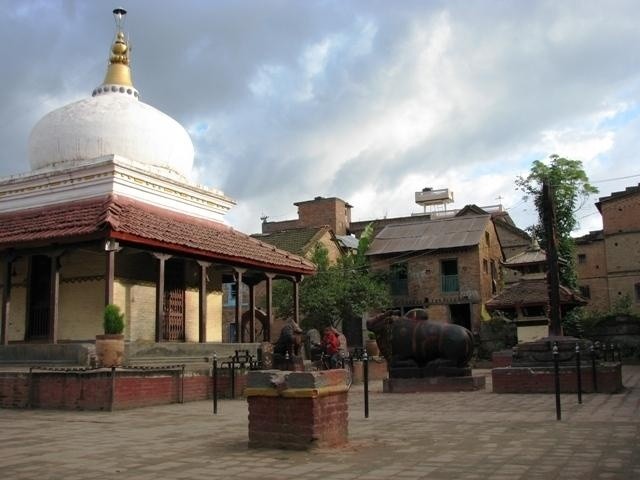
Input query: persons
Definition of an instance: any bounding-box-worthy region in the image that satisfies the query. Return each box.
[316,326,341,369]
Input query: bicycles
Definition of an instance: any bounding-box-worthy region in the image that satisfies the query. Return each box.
[311,347,353,392]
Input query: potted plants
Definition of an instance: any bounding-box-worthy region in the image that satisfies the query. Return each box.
[365,331,380,357]
[95,305,126,369]
